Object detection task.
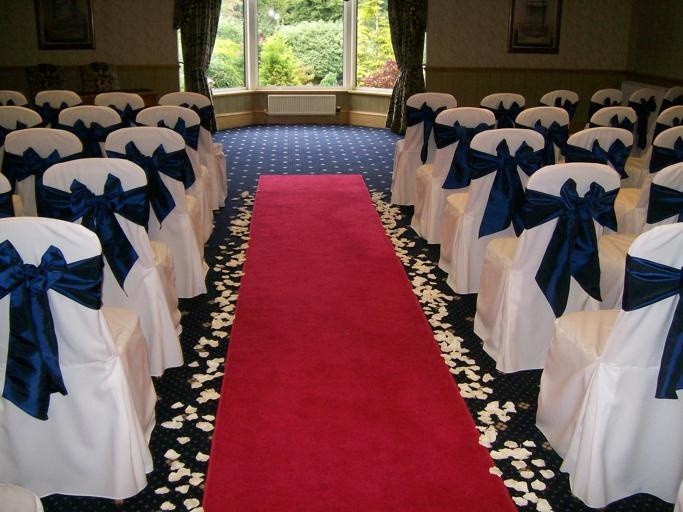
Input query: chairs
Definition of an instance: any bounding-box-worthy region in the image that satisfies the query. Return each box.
[390,91,458,209]
[472,163,622,374]
[481,85,683,310]
[437,127,545,293]
[410,108,497,246]
[0,215,161,502]
[43,159,185,375]
[104,127,212,301]
[531,221,683,511]
[75,62,119,90]
[24,61,67,107]
[0,90,226,232]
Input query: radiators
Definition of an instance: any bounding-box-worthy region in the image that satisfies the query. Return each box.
[266,93,336,117]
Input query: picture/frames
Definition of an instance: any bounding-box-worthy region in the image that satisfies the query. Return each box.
[505,0,564,55]
[33,2,97,51]
